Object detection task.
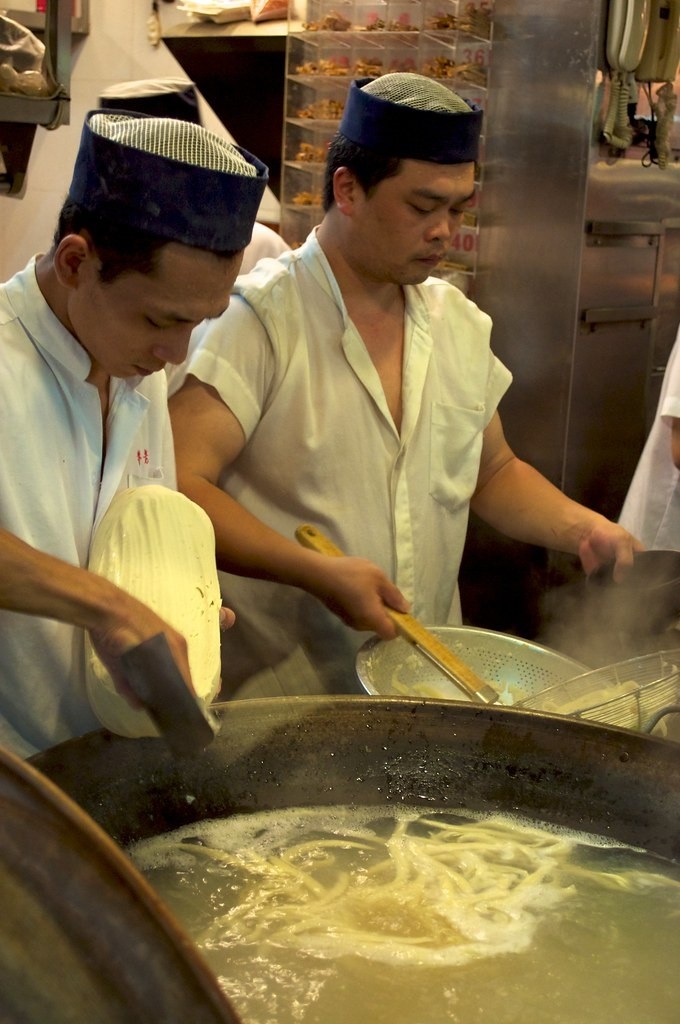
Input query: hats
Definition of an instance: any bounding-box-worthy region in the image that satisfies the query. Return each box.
[69,108,270,253]
[98,78,202,128]
[337,71,484,164]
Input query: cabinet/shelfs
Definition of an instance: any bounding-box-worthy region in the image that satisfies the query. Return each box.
[279,31,494,298]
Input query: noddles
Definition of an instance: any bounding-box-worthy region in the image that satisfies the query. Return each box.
[127,804,680,1024]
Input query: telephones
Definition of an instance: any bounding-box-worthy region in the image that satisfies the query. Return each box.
[606,0,680,84]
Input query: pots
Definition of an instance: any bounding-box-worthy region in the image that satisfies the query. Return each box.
[24,706,680,1024]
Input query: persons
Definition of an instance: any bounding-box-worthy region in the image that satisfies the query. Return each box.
[167,73,645,697]
[0,107,271,761]
[98,75,293,276]
[620,320,680,556]
